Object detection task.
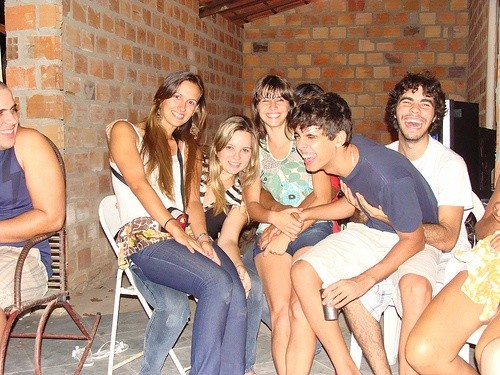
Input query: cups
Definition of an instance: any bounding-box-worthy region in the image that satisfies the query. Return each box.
[319,289,339,321]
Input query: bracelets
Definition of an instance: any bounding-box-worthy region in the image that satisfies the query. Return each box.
[164,218,175,229]
[197,233,206,241]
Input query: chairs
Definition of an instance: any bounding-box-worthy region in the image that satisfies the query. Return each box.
[0,134,101,375]
[98,196,488,375]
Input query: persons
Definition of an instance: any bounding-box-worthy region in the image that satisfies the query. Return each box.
[104,71,500,375]
[0,81,65,341]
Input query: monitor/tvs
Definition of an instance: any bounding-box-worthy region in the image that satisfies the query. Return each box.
[430,98,497,200]
